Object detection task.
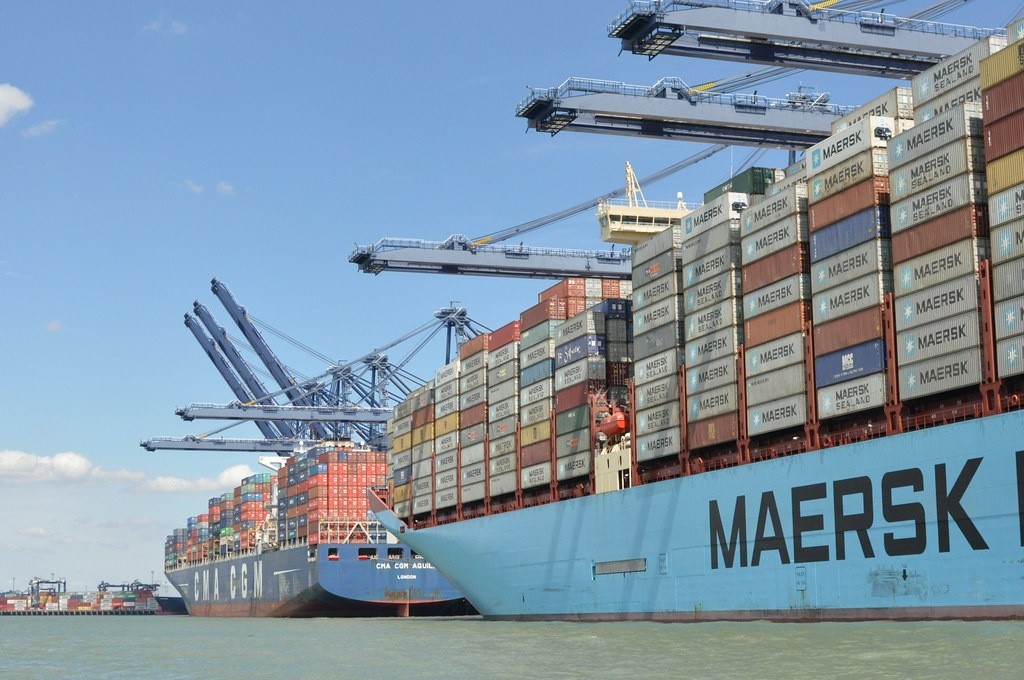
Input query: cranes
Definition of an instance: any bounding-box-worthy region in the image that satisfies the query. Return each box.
[138,1,1005,457]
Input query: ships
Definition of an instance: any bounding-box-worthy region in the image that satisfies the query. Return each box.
[363,253,1023,624]
[163,506,482,620]
